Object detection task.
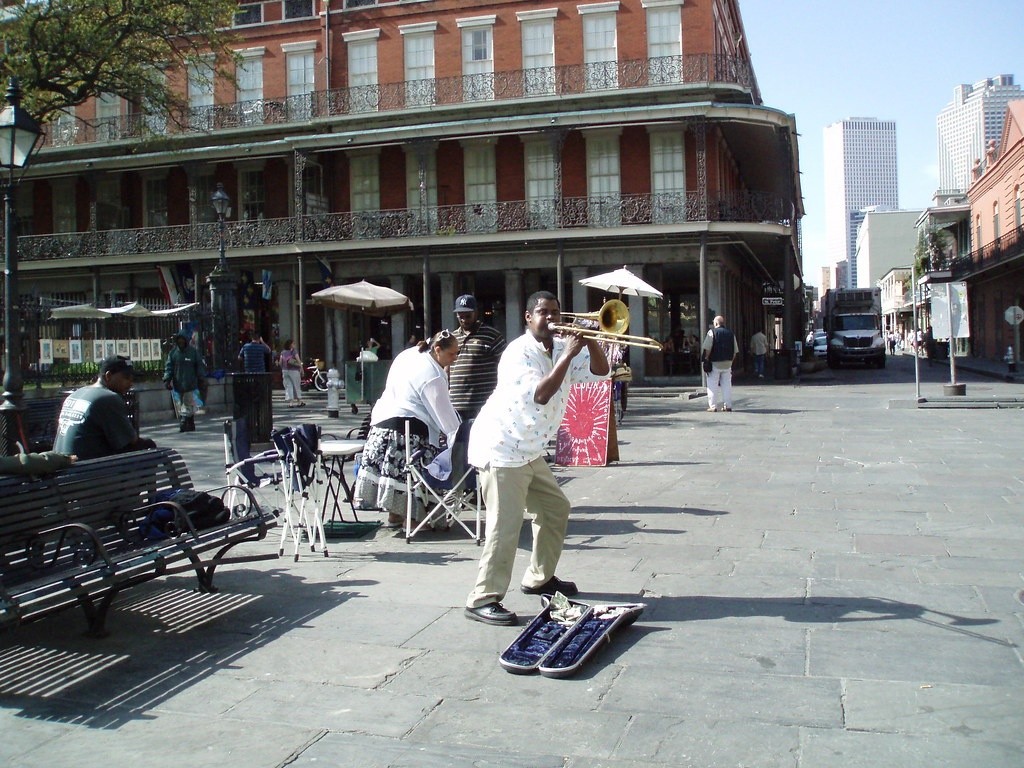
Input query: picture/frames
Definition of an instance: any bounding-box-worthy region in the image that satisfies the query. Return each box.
[39,338,162,364]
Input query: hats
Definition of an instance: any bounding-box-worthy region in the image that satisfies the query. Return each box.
[453,294,477,312]
[102,355,138,377]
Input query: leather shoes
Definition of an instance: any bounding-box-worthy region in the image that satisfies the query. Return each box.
[521,575,578,595]
[466,601,518,626]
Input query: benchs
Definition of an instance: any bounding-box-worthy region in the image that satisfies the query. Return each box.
[0,449,279,642]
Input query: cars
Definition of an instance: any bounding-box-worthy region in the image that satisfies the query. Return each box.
[813,328,827,359]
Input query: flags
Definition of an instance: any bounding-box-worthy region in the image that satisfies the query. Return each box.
[156,264,180,306]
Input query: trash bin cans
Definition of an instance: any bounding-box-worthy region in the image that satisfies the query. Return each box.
[226,371,282,442]
[771,347,799,380]
[934,342,949,360]
[345,360,390,404]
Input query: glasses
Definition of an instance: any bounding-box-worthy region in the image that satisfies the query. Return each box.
[434,328,453,344]
[457,313,473,319]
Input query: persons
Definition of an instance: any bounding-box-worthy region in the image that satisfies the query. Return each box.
[278,339,307,408]
[466,291,613,627]
[441,294,508,510]
[885,327,940,367]
[352,330,462,532]
[700,315,739,411]
[748,328,766,376]
[806,330,815,358]
[160,327,207,432]
[236,332,272,373]
[648,334,700,377]
[51,355,157,498]
[0,451,79,479]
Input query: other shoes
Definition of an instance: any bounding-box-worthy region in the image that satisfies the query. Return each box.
[707,408,717,412]
[469,493,484,505]
[721,407,731,412]
[180,422,195,432]
[754,373,758,378]
[760,377,763,381]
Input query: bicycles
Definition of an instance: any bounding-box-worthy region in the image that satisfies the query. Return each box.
[301,357,329,391]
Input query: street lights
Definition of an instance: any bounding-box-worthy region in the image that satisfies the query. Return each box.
[204,182,240,371]
[0,73,46,458]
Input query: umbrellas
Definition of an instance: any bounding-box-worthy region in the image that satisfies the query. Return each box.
[576,265,664,303]
[312,279,413,400]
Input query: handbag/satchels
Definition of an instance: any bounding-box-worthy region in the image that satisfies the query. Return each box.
[703,360,712,375]
[287,350,301,369]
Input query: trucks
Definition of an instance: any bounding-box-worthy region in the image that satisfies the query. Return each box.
[820,287,886,370]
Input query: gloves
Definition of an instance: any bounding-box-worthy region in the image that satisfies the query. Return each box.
[198,376,204,384]
[164,381,173,390]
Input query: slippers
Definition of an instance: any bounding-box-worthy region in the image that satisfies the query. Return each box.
[403,520,433,532]
[297,402,305,407]
[388,518,403,527]
[287,405,294,408]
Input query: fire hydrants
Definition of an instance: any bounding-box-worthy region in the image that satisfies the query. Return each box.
[1004,346,1017,372]
[324,369,345,419]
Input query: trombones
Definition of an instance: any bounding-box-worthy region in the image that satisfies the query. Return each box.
[546,300,665,352]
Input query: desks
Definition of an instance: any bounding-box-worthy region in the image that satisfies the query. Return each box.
[322,440,366,524]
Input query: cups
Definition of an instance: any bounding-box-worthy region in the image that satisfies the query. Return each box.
[370,338,373,342]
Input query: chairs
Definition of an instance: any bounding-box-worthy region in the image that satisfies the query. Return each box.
[272,419,332,562]
[403,418,483,547]
[218,416,306,525]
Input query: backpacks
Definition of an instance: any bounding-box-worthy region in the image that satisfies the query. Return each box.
[133,488,231,541]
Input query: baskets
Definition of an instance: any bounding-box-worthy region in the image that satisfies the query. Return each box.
[315,362,324,369]
